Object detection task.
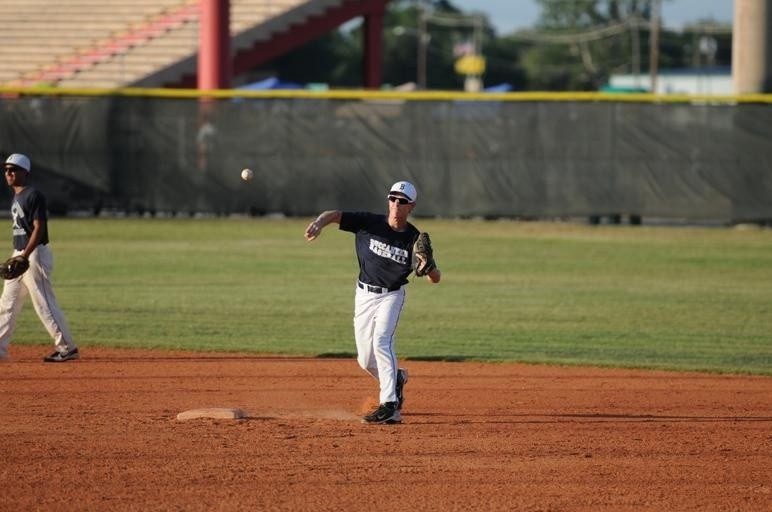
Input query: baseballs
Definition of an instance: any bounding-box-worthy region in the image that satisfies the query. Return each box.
[242,168,254,180]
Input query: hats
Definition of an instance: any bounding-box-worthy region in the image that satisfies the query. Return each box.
[387,179,418,204]
[0,152,32,175]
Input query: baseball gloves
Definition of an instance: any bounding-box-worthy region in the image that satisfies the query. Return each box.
[412,232,434,277]
[1,255,28,279]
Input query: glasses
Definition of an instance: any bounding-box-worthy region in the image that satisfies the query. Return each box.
[0,165,19,173]
[386,194,415,206]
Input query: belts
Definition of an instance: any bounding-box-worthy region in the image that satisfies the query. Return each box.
[356,278,400,294]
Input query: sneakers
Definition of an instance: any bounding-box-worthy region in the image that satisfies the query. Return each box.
[394,365,409,411]
[361,400,404,426]
[43,347,80,363]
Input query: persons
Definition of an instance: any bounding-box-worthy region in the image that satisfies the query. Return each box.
[304,181,440,424]
[0,154,80,362]
